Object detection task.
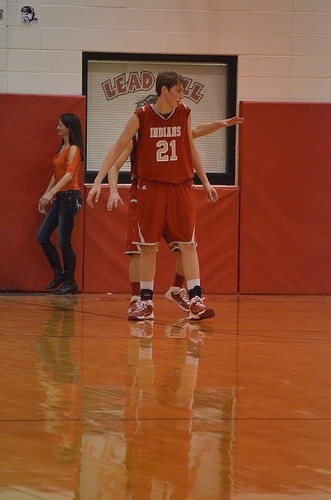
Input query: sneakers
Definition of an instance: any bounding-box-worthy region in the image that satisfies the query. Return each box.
[165,286,191,312]
[128,295,141,314]
[188,296,215,321]
[128,299,154,320]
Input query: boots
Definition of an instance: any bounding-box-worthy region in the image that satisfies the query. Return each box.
[53,254,78,295]
[45,251,66,289]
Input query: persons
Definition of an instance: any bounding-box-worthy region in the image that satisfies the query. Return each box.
[87,70,244,320]
[35,112,86,295]
